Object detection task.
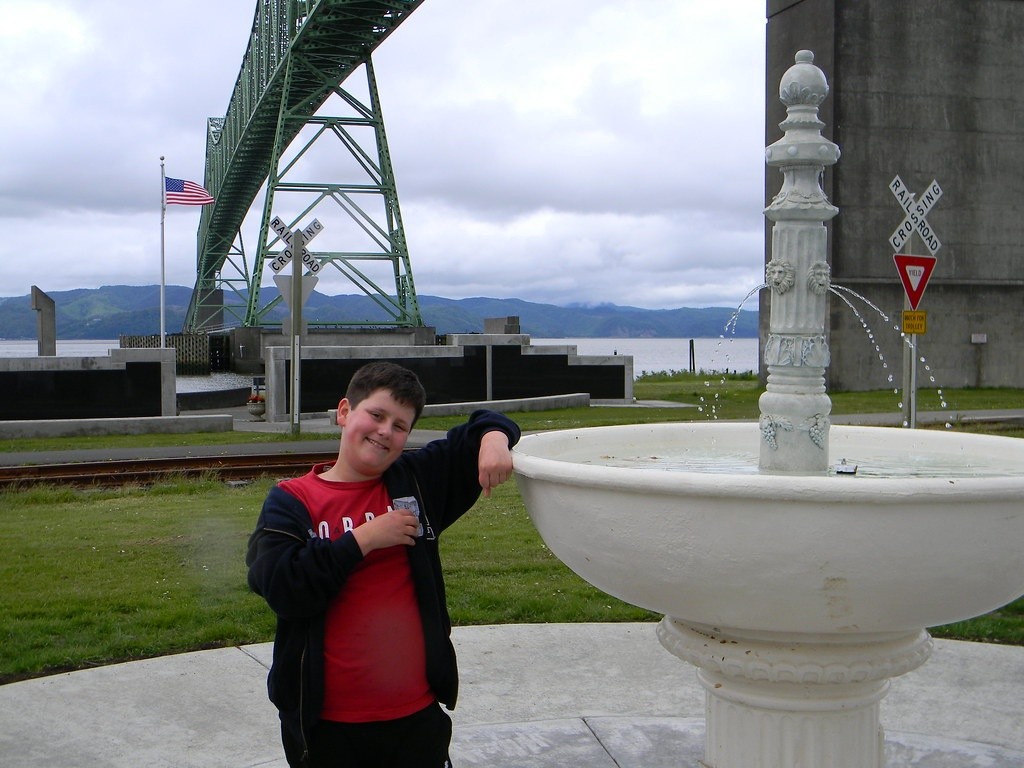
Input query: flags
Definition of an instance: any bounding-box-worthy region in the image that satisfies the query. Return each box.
[165,177,214,206]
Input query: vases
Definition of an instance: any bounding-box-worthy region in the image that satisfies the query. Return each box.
[247,403,266,422]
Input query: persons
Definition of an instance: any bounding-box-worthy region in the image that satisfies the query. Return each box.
[246,361,522,768]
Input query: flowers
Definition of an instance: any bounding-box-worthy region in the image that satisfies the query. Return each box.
[248,395,265,403]
